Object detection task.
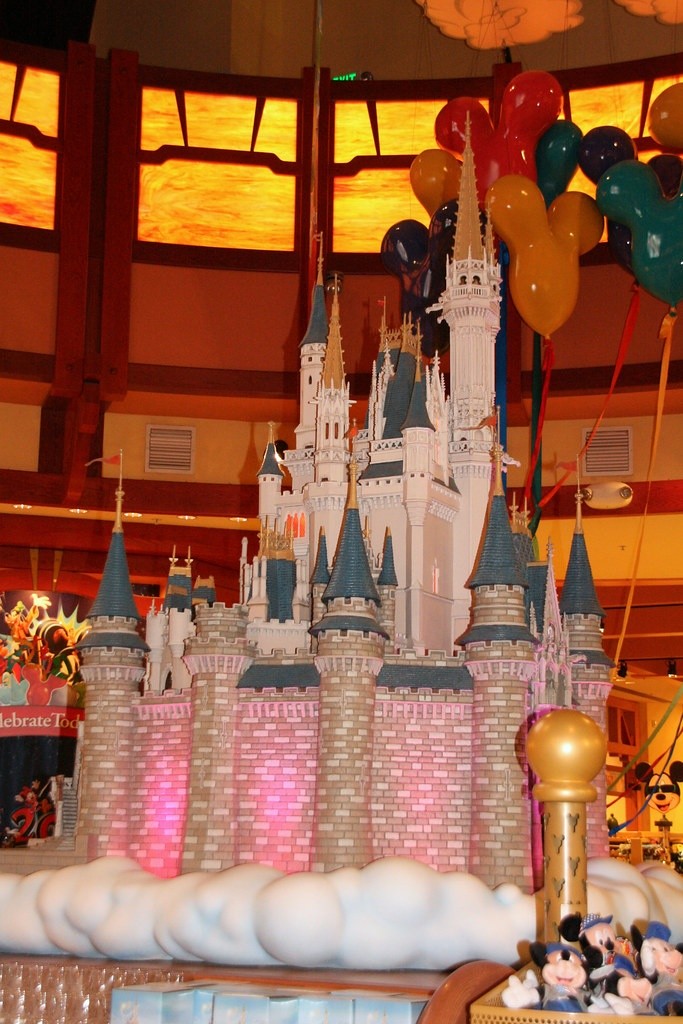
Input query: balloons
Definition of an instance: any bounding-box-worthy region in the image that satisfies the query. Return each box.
[533,119,585,215]
[410,149,463,218]
[594,158,683,316]
[381,197,488,359]
[576,126,683,286]
[433,69,561,214]
[647,80,683,157]
[485,174,604,343]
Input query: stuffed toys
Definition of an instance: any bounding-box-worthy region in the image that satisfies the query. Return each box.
[498,911,683,1019]
